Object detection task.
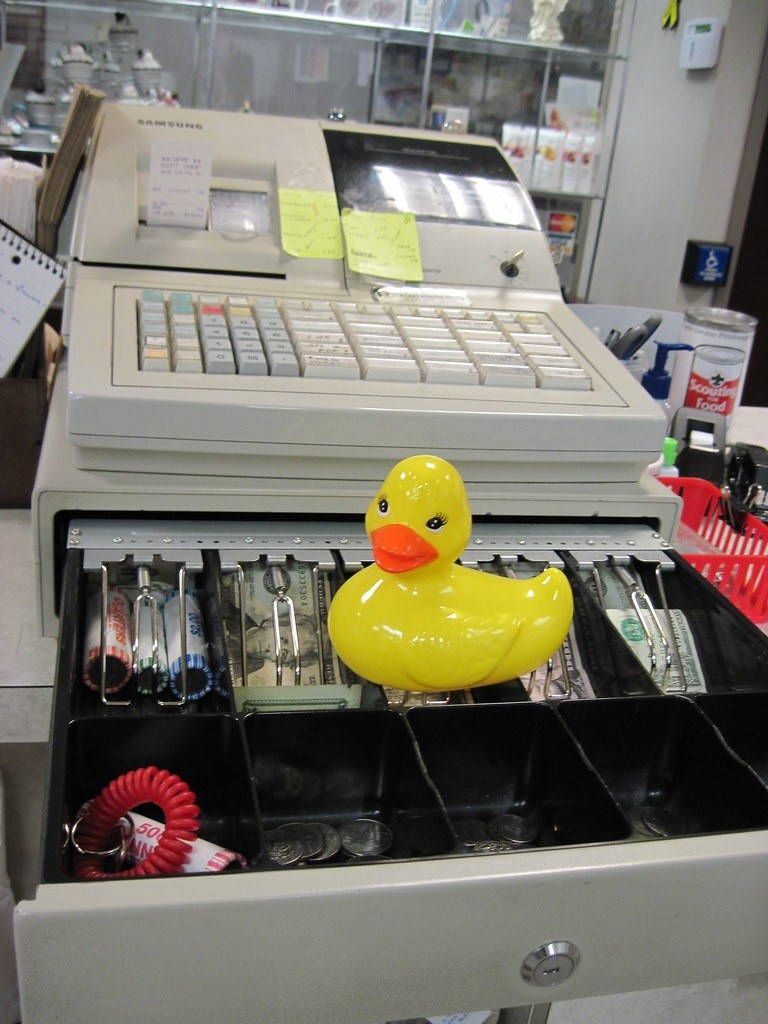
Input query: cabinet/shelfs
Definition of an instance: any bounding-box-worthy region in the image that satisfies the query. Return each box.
[0,1,638,300]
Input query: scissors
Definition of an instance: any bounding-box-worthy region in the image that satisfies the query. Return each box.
[610,312,663,360]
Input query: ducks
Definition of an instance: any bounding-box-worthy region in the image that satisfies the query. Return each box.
[326,455,575,692]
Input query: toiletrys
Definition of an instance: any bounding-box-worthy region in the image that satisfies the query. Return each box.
[640,334,696,448]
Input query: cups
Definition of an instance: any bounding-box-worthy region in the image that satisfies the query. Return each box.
[25,96,53,129]
[134,66,163,98]
[666,306,756,438]
[97,67,119,92]
[108,26,136,52]
[59,59,93,87]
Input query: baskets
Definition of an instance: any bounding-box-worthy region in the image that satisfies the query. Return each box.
[655,476,768,623]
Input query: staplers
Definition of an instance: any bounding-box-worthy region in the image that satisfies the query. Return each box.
[725,441,768,523]
